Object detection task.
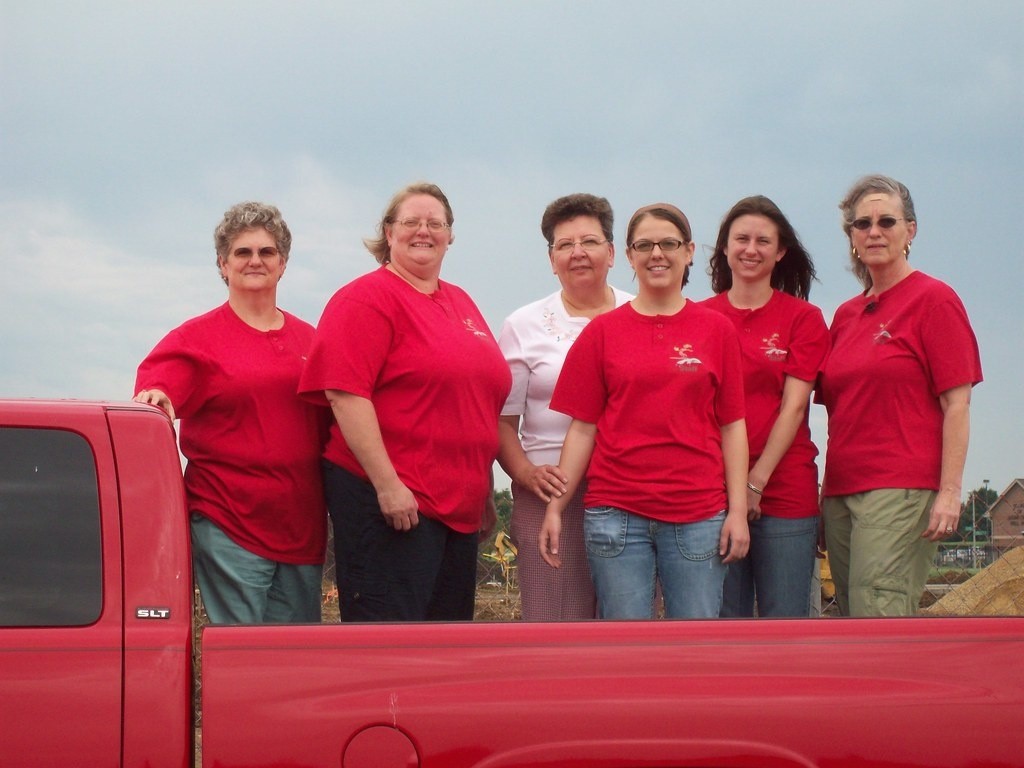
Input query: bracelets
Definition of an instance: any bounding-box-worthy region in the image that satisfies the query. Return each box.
[747,482,762,494]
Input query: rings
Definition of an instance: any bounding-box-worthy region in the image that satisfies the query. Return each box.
[143,390,146,391]
[947,528,952,532]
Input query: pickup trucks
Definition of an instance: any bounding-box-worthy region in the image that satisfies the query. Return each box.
[0,399,1024,768]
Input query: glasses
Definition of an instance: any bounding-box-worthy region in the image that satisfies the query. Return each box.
[629,238,688,253]
[549,236,608,256]
[233,246,279,259]
[391,215,451,233]
[849,215,910,230]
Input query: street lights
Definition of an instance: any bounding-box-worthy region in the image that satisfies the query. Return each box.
[982,478,989,535]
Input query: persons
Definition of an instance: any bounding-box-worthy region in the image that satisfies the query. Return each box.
[697,196,827,618]
[296,184,512,621]
[496,193,637,622]
[132,201,327,626]
[810,176,983,619]
[537,203,750,621]
